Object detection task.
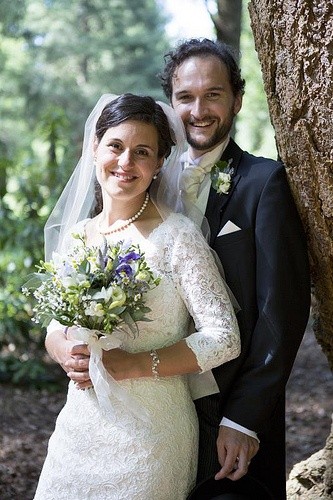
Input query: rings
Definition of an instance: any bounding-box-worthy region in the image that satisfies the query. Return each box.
[248,461,251,465]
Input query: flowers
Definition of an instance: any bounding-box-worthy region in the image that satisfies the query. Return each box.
[20,228,162,341]
[210,158,234,194]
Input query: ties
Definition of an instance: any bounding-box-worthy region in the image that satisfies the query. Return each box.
[179,162,215,217]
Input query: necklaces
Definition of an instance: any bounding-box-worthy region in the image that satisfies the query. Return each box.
[97,192,149,236]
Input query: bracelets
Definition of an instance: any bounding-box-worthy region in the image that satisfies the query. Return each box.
[150,349,161,381]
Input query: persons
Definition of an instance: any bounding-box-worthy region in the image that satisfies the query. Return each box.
[158,39,311,500]
[23,94,240,500]
